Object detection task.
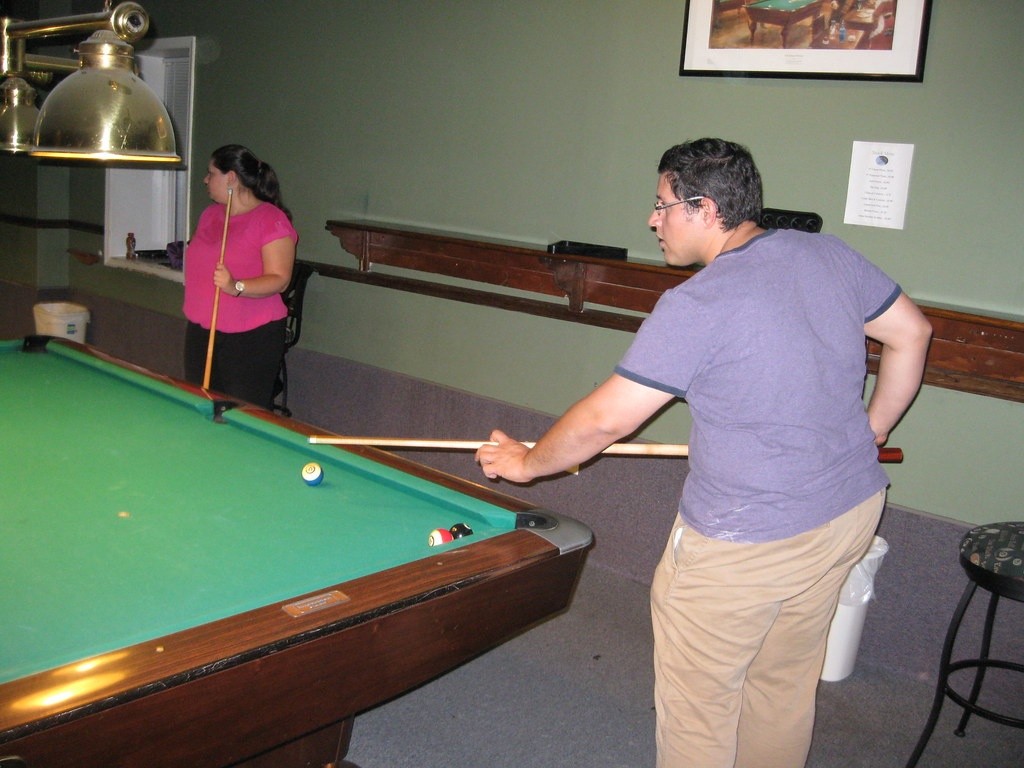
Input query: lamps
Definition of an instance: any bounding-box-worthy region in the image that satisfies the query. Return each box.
[0,0,182,163]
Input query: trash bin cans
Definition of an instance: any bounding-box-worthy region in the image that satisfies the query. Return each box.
[819,535,891,683]
[33,302,90,344]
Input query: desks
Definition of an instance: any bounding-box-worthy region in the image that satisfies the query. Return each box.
[840,8,876,36]
[745,0,823,47]
[0,335,594,768]
[808,27,866,49]
[713,0,745,28]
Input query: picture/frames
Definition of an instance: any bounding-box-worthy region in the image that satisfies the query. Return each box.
[679,0,934,84]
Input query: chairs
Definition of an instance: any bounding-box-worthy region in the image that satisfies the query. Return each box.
[812,15,825,41]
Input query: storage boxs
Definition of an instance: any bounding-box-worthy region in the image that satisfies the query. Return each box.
[34,302,90,344]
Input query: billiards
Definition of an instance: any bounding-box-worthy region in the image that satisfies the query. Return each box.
[301,462,324,486]
[449,523,474,540]
[428,528,454,547]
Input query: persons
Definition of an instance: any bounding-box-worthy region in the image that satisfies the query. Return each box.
[472,138,937,767]
[181,144,301,411]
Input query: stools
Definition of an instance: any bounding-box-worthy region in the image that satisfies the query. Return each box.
[906,522,1024,768]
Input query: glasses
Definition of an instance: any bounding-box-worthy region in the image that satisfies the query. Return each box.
[653,196,706,216]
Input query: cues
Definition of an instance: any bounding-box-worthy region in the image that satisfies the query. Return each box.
[203,188,234,391]
[307,435,905,465]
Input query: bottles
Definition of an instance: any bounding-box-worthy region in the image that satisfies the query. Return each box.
[126,233,136,260]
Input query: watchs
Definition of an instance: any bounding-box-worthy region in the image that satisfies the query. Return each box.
[234,279,244,298]
